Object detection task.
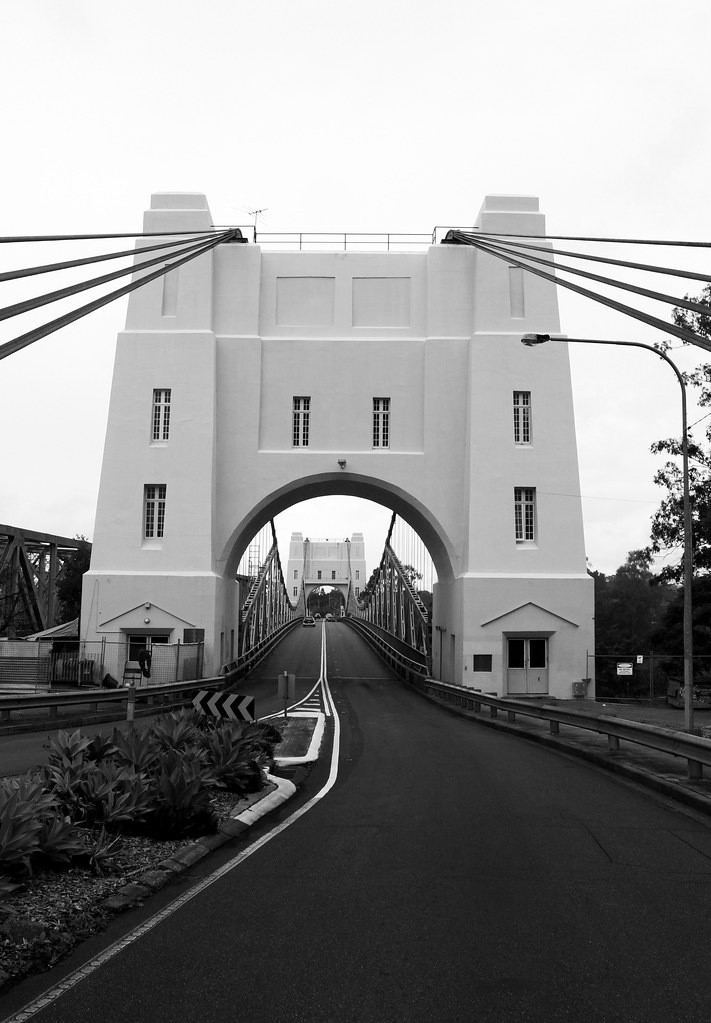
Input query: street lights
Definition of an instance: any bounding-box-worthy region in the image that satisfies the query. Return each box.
[522,332,693,733]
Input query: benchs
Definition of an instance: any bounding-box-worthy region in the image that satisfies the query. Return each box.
[122,668,142,686]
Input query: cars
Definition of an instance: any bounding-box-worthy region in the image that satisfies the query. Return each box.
[302,615,316,628]
[326,614,335,622]
[314,613,321,619]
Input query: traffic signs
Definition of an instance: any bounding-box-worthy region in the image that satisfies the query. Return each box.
[192,691,256,722]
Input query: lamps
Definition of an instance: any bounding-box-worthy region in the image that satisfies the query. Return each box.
[337,459,345,466]
[144,617,149,624]
